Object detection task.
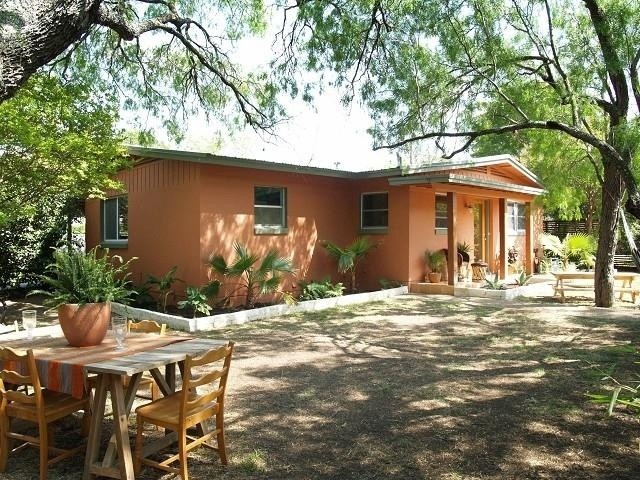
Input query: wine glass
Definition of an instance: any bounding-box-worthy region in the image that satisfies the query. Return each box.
[112,317,129,352]
[22,310,36,341]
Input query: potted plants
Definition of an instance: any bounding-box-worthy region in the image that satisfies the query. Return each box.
[507,245,519,274]
[424,249,446,283]
[22,241,141,347]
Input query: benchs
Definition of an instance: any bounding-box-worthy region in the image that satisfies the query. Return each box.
[546,282,640,304]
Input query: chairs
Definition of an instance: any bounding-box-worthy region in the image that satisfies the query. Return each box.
[439,247,469,282]
[0,318,236,480]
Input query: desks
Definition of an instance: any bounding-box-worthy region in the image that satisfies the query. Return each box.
[549,269,640,304]
